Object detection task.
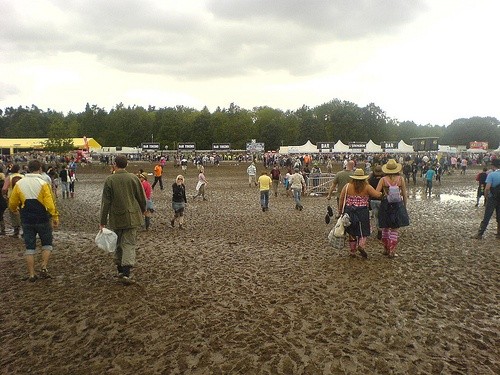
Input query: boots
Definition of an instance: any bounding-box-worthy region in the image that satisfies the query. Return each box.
[389,238,399,259]
[358,235,368,257]
[71,191,74,198]
[62,192,65,199]
[349,241,357,256]
[145,216,150,231]
[470,220,488,239]
[67,191,70,199]
[382,238,389,255]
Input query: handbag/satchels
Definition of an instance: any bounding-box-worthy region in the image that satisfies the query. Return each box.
[333,214,351,237]
[95,228,118,252]
[328,228,344,249]
[67,176,71,182]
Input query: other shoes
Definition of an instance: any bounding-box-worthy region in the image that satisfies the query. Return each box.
[295,203,300,210]
[300,206,303,211]
[119,272,136,285]
[172,219,185,230]
[262,206,266,212]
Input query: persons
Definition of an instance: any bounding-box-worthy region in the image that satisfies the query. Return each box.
[263,153,321,196]
[99,154,115,165]
[291,168,306,211]
[257,171,272,212]
[323,153,468,196]
[138,169,154,230]
[470,152,500,239]
[112,167,115,174]
[151,162,164,190]
[327,158,410,259]
[97,155,148,278]
[8,159,59,281]
[0,150,75,164]
[0,162,24,237]
[155,154,167,170]
[174,152,264,175]
[169,175,187,229]
[192,169,207,201]
[247,162,258,187]
[43,160,78,199]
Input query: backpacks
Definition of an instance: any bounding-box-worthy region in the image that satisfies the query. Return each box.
[11,176,22,189]
[382,176,401,202]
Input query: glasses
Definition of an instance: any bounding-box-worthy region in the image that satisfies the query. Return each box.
[178,178,183,180]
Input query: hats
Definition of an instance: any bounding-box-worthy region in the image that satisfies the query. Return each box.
[381,159,402,173]
[349,169,368,179]
[374,165,384,175]
